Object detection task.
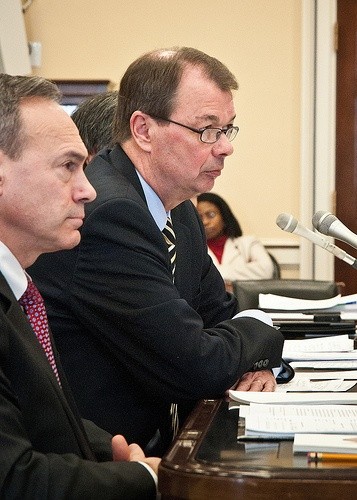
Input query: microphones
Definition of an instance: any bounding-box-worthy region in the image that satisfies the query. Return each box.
[275,212,357,271]
[312,209,357,250]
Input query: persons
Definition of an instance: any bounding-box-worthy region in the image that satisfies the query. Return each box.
[70,92,119,169]
[0,73,161,500]
[24,46,295,457]
[196,193,275,292]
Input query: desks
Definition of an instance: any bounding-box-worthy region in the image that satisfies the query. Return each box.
[157,319,357,500]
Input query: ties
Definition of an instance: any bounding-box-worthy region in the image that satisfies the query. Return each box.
[162,217,178,284]
[20,281,61,390]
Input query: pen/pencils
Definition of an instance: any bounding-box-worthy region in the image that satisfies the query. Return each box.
[307,452,357,461]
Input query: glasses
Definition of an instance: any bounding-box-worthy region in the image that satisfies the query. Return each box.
[150,113,240,144]
[199,208,222,221]
